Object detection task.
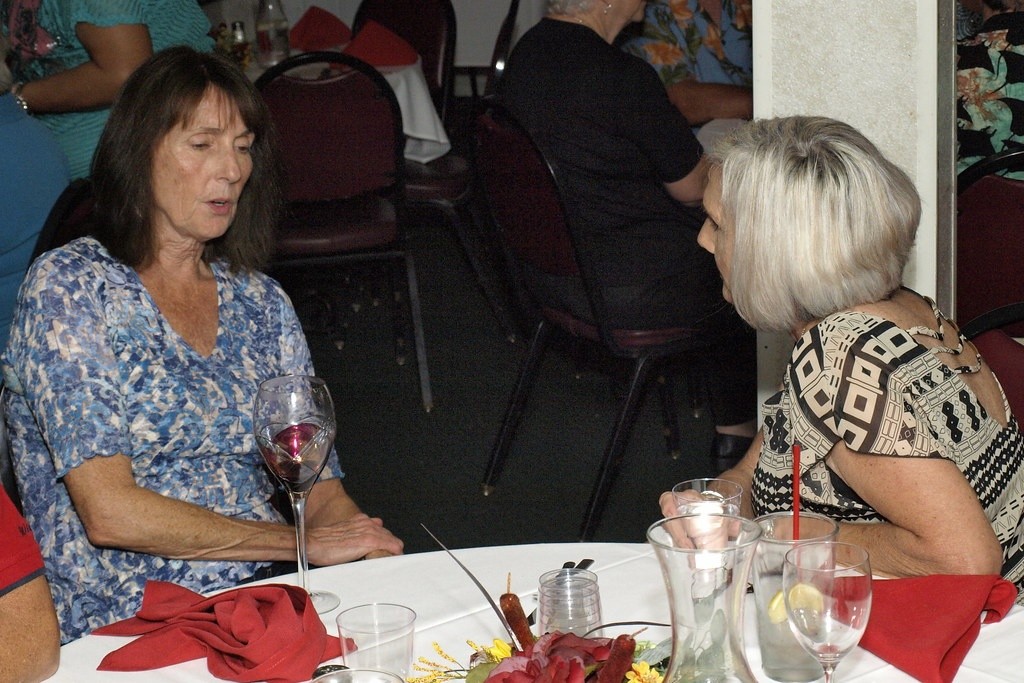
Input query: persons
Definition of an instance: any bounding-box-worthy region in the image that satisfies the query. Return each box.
[957,0,1024,201]
[0,0,218,385]
[659,116,1024,604]
[0,43,406,647]
[492,0,753,474]
[0,481,59,682]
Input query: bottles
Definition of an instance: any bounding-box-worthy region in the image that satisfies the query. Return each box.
[255,0,289,68]
[215,22,251,42]
[647,513,758,683]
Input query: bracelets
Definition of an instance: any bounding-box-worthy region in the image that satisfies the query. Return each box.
[15,85,30,114]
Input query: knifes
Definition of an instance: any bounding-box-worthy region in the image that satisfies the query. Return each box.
[525,559,594,626]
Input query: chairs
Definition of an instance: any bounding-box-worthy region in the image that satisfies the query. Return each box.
[402,0,522,342]
[253,51,433,414]
[467,102,746,543]
[352,0,458,134]
[957,146,1024,437]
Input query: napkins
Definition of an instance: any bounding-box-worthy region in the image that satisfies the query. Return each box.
[289,6,349,51]
[829,573,1018,683]
[90,580,357,683]
[341,20,417,66]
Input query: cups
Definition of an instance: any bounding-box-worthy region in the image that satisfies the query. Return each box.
[306,668,405,683]
[672,479,743,518]
[336,603,417,680]
[751,511,839,681]
[537,569,602,642]
[233,38,255,77]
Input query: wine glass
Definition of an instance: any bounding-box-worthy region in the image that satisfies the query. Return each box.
[783,542,872,683]
[253,374,339,615]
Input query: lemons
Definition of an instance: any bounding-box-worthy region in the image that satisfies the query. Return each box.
[769,584,824,623]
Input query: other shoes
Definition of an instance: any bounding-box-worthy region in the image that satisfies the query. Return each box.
[711,432,755,472]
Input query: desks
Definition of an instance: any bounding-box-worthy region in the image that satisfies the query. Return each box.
[42,542,1024,683]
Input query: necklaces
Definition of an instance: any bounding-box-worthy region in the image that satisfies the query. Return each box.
[569,16,594,29]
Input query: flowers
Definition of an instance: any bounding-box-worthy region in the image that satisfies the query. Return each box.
[212,31,248,65]
[407,594,668,683]
